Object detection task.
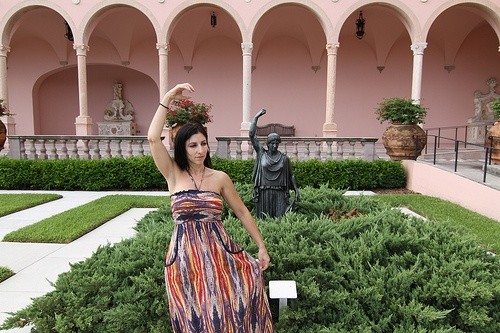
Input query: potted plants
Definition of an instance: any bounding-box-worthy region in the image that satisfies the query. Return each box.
[484,94,500,165]
[373,96,430,164]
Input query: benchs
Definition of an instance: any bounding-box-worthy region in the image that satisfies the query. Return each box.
[256,123,296,146]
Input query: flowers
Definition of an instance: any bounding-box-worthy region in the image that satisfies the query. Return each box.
[165,95,215,130]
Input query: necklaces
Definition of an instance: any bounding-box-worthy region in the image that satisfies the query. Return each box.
[187,166,205,190]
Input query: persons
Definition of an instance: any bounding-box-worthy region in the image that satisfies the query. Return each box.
[249,108,300,219]
[146,80,273,333]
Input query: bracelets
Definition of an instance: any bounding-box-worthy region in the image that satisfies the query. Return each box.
[160,102,170,109]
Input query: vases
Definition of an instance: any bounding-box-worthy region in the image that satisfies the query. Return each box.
[170,124,208,141]
[0,120,7,152]
